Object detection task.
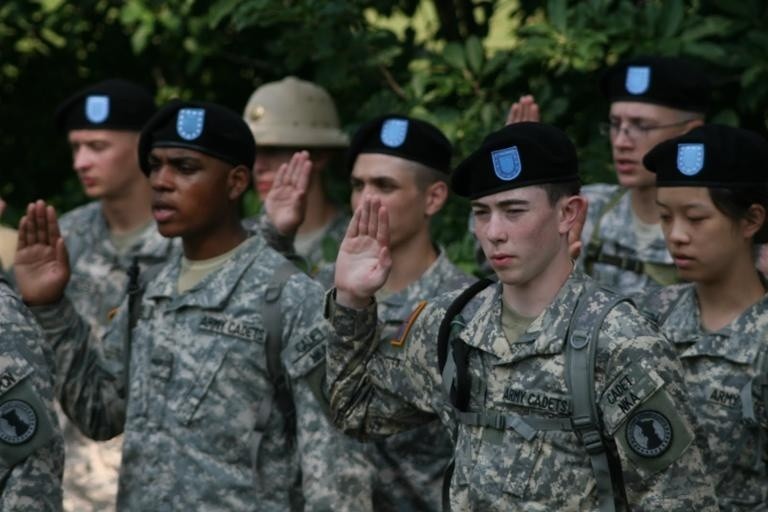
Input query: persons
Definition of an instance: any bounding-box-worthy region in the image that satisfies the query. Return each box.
[0,57,768,512]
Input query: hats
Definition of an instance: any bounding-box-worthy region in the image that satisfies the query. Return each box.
[51,80,158,140]
[643,124,768,188]
[597,56,741,114]
[138,98,256,178]
[339,114,452,179]
[243,75,350,150]
[450,122,581,201]
